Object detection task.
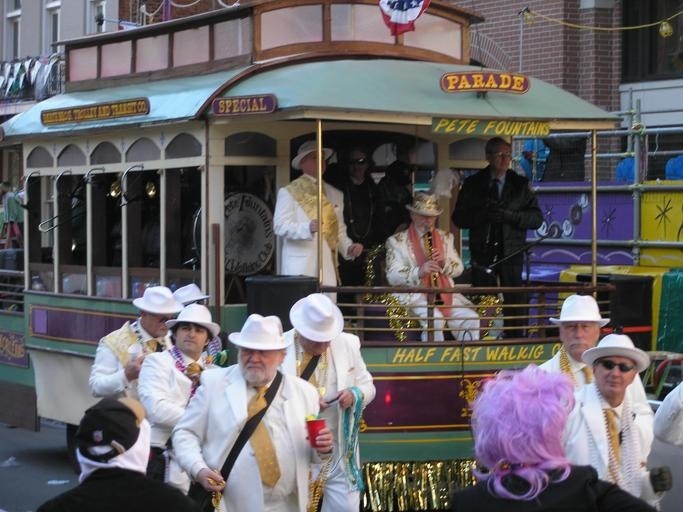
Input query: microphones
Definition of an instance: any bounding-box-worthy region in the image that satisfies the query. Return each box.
[471,259,493,275]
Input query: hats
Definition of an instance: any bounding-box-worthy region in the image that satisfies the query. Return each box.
[76,397,145,463]
[133,282,343,351]
[549,295,610,327]
[291,140,332,169]
[405,191,443,216]
[581,334,649,373]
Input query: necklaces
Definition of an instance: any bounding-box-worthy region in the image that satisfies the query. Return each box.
[294,333,328,399]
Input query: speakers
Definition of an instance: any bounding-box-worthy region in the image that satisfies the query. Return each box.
[245,276,317,332]
[576,272,654,351]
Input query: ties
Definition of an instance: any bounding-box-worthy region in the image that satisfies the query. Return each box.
[247,388,280,487]
[581,366,592,384]
[489,179,498,200]
[300,352,315,387]
[604,409,620,485]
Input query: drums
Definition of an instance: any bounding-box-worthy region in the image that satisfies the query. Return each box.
[190,191,276,277]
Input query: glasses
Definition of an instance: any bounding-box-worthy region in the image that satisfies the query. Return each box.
[598,361,634,371]
[495,152,510,157]
[349,158,365,165]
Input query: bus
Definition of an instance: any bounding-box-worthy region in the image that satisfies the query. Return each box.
[1,0,623,510]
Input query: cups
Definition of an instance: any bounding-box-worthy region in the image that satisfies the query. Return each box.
[305,419,325,448]
[127,343,143,361]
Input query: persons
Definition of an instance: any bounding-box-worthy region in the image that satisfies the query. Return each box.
[88,286,183,396]
[335,147,381,284]
[376,161,415,236]
[563,334,672,507]
[385,191,480,341]
[274,140,362,303]
[451,138,542,339]
[173,283,223,366]
[535,295,655,456]
[34,396,198,511]
[654,381,683,446]
[279,293,375,512]
[171,312,332,511]
[137,304,223,494]
[445,368,658,511]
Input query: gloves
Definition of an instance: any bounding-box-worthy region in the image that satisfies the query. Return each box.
[476,200,512,224]
